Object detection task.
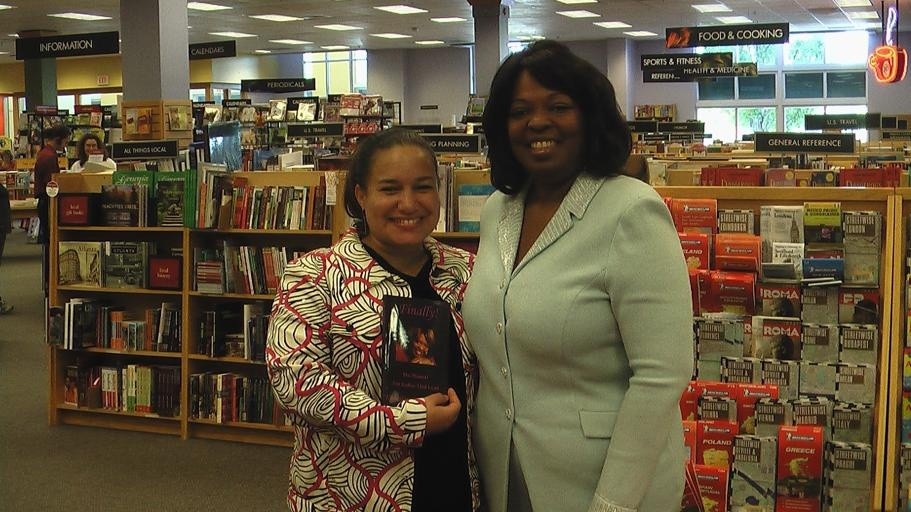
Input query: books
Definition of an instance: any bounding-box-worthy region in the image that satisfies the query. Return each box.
[53,149,197,292]
[435,157,499,235]
[383,294,453,405]
[193,160,336,295]
[618,114,911,510]
[43,294,298,427]
[0,104,113,201]
[192,93,395,172]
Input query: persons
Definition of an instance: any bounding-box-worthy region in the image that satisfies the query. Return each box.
[264,132,477,511]
[2,185,15,314]
[394,323,434,365]
[30,124,71,295]
[461,41,696,509]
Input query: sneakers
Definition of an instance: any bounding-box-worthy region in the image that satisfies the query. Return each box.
[0,296,14,315]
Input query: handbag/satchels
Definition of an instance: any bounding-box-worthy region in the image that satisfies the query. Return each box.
[23,216,43,245]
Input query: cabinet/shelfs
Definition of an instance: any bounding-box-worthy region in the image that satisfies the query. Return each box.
[1,96,121,219]
[49,156,652,448]
[192,98,401,172]
[652,187,911,512]
